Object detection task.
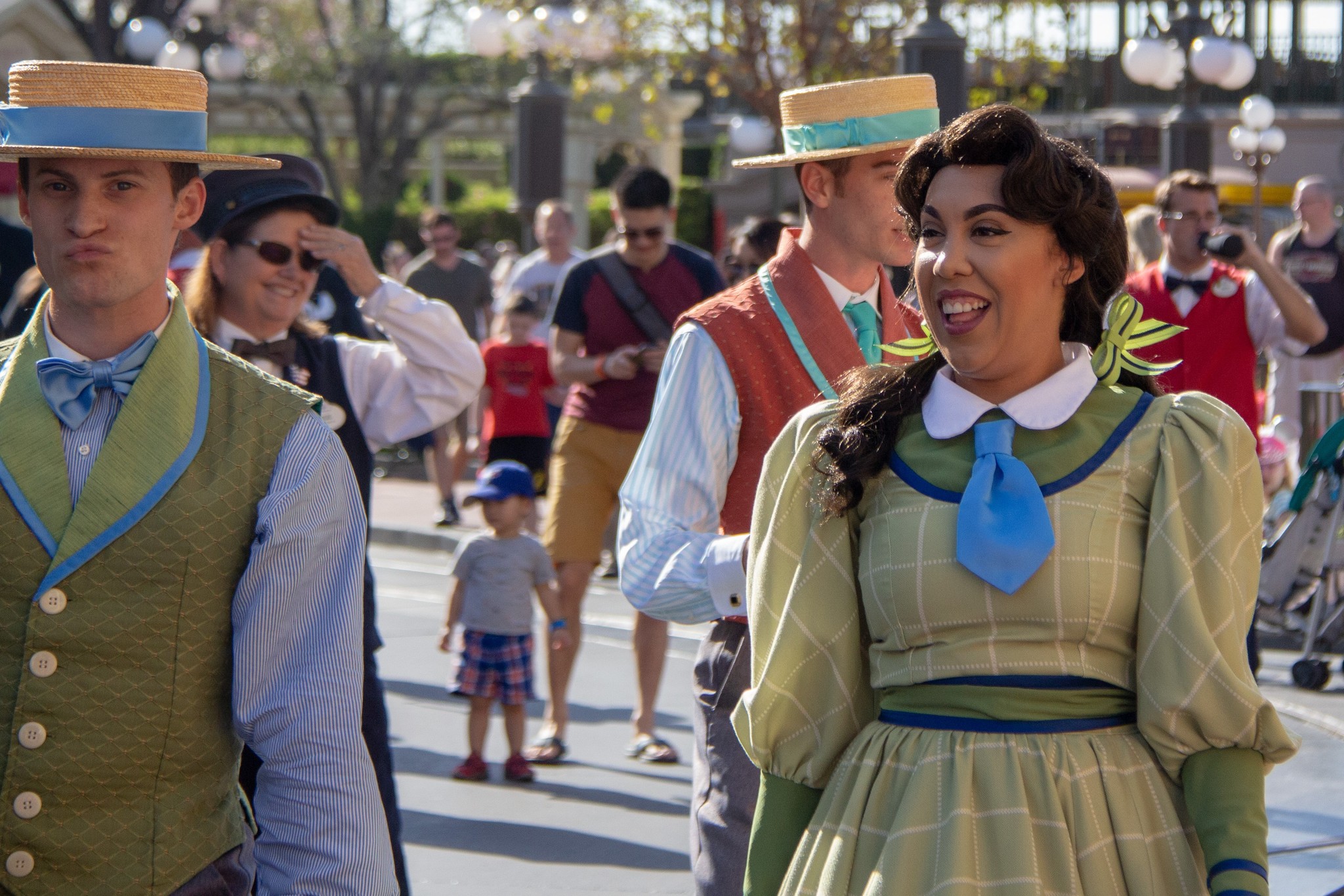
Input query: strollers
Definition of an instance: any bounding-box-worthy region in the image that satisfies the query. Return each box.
[1249,415,1343,696]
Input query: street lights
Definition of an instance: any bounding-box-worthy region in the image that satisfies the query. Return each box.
[1125,0,1256,202]
[463,4,603,243]
[1227,96,1286,254]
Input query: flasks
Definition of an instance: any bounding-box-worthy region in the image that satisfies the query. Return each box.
[1197,231,1243,260]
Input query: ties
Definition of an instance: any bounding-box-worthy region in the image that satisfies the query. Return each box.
[956,419,1055,596]
[840,300,883,366]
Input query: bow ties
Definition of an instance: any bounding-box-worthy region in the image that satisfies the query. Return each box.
[1165,275,1210,296]
[230,338,296,367]
[35,329,159,431]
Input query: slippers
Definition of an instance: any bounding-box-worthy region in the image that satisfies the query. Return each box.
[624,739,677,763]
[522,736,567,764]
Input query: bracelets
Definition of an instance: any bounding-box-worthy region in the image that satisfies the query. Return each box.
[548,618,567,633]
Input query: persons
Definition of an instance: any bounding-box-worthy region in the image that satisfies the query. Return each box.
[727,215,792,291]
[538,163,733,774]
[1251,428,1300,551]
[181,150,495,895]
[297,183,607,547]
[435,466,581,795]
[1256,174,1343,466]
[1,57,408,889]
[1100,157,1329,683]
[724,101,1308,893]
[614,73,941,896]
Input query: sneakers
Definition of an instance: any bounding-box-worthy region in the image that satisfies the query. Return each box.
[505,755,534,780]
[451,755,488,781]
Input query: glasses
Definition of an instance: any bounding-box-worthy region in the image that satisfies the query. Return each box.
[621,226,665,239]
[1161,209,1224,225]
[232,237,326,272]
[723,255,761,281]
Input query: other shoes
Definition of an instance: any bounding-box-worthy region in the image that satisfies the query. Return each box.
[437,511,459,525]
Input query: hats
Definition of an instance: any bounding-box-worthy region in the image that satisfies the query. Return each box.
[462,458,535,506]
[731,74,943,170]
[190,152,341,243]
[0,58,285,172]
